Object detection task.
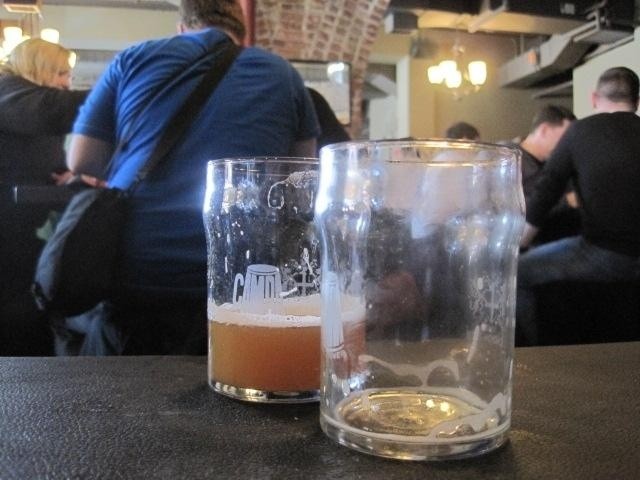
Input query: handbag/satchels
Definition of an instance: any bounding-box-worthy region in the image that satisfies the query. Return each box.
[31,188,129,317]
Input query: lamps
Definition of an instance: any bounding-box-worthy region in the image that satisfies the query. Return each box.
[1,12,76,67]
[427,32,488,101]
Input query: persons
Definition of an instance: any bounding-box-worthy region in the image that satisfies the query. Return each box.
[467,101,582,257]
[514,65,640,345]
[2,35,105,355]
[64,1,323,356]
[406,122,483,345]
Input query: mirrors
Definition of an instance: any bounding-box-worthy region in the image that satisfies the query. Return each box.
[286,59,352,127]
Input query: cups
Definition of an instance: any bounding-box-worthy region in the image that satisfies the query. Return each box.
[319,141,527,464]
[202,158,365,405]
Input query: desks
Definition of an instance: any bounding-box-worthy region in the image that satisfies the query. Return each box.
[0,342,639,479]
